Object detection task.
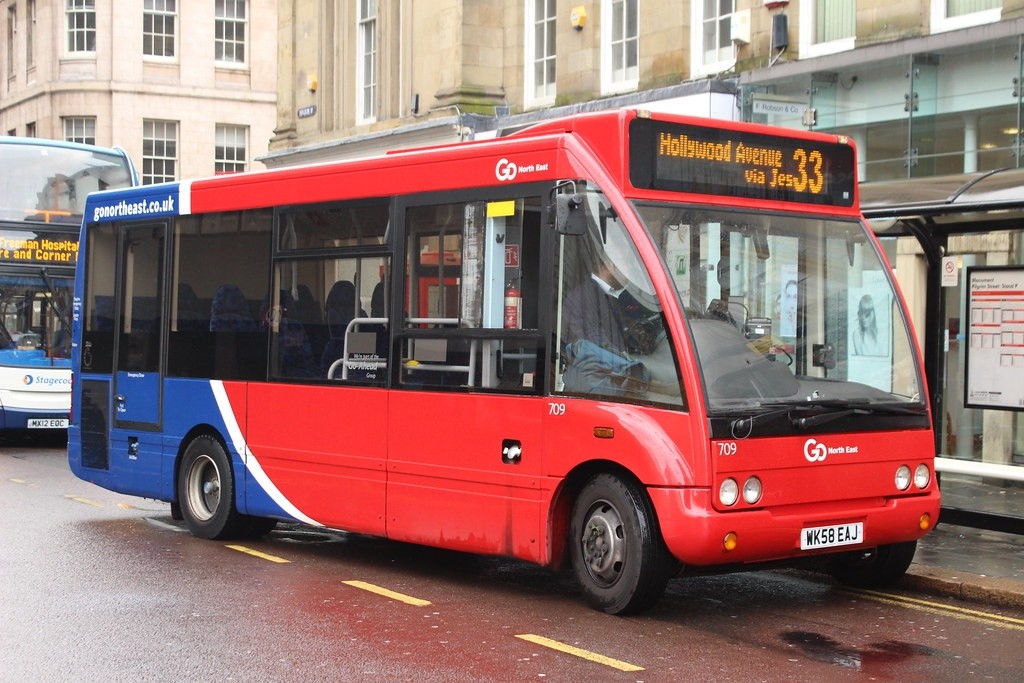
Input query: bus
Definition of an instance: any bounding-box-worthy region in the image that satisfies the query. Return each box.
[65,107,944,617]
[0,136,138,446]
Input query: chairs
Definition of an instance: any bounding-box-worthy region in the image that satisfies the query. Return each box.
[92,274,387,383]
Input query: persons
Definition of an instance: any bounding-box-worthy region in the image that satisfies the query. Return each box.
[852,295,882,356]
[560,238,633,379]
[785,279,798,331]
